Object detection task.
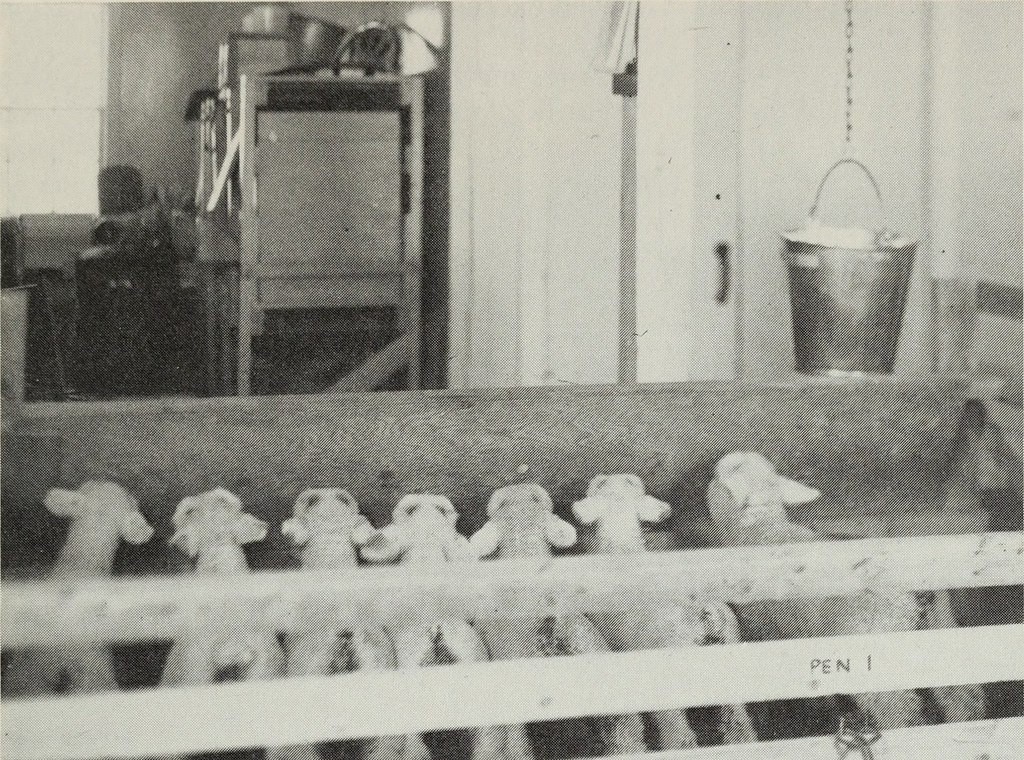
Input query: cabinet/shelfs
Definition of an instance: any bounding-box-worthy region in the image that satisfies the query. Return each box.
[195,77,426,399]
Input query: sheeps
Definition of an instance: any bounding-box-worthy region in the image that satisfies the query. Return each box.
[10,478,156,691]
[467,482,648,760]
[155,488,287,684]
[570,472,759,747]
[704,449,986,728]
[360,494,505,760]
[266,485,407,760]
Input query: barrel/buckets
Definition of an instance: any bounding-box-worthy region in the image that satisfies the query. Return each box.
[780,156,916,380]
